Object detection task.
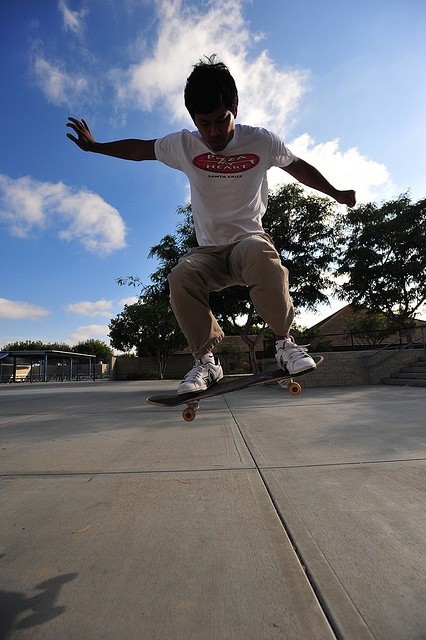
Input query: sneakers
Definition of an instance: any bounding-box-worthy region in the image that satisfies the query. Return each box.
[275,334,318,377]
[177,356,225,398]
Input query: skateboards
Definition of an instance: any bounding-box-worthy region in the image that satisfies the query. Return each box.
[146,356,324,422]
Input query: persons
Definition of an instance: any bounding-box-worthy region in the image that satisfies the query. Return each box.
[64,51,358,394]
